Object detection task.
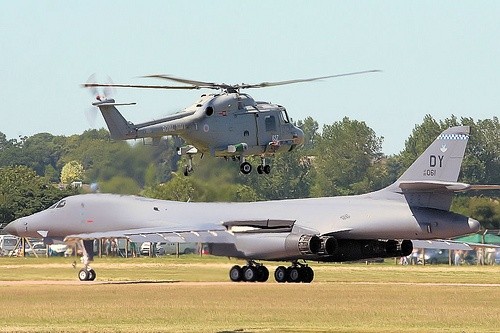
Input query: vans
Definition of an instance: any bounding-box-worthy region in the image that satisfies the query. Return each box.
[24,242,46,258]
[141,242,197,256]
[1,237,18,255]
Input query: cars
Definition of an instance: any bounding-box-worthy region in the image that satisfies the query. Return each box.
[406,248,454,266]
[49,244,68,256]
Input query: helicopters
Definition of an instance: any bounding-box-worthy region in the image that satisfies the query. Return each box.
[81,69,384,176]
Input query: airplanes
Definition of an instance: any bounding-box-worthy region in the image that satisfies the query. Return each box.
[1,124,482,284]
